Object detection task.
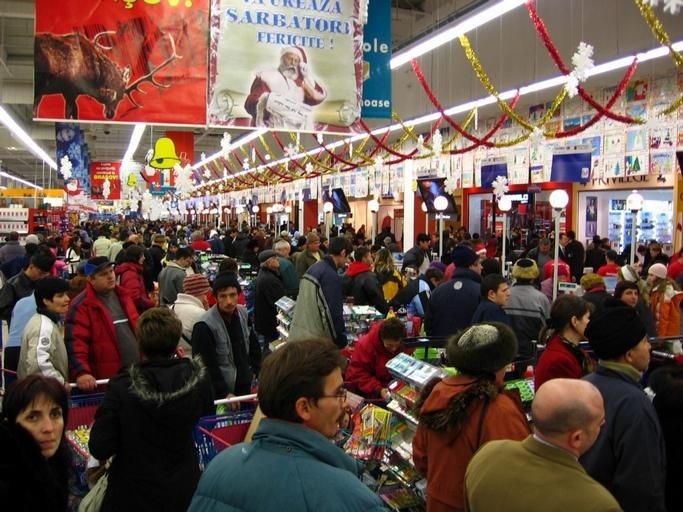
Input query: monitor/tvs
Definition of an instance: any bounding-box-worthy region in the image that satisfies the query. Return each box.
[324,187,351,214]
[416,177,457,215]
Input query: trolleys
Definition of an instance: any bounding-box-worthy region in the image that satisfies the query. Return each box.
[186,390,380,492]
[64,378,108,479]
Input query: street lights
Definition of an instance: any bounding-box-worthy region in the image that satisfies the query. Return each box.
[164,202,293,234]
[433,194,449,263]
[320,203,335,243]
[547,187,571,304]
[367,200,381,248]
[624,186,644,271]
[497,196,515,277]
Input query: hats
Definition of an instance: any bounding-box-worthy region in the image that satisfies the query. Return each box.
[452,243,487,268]
[258,250,277,262]
[580,264,647,360]
[648,263,667,279]
[512,258,540,279]
[308,232,320,242]
[281,45,307,64]
[183,274,210,296]
[84,257,118,276]
[446,321,517,372]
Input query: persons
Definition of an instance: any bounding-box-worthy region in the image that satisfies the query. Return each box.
[245,46,328,127]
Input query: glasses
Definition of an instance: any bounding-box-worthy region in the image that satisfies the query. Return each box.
[321,389,347,402]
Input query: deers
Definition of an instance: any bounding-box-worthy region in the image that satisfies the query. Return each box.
[34,30,184,120]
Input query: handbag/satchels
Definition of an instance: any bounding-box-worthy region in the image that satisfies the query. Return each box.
[78,472,110,512]
